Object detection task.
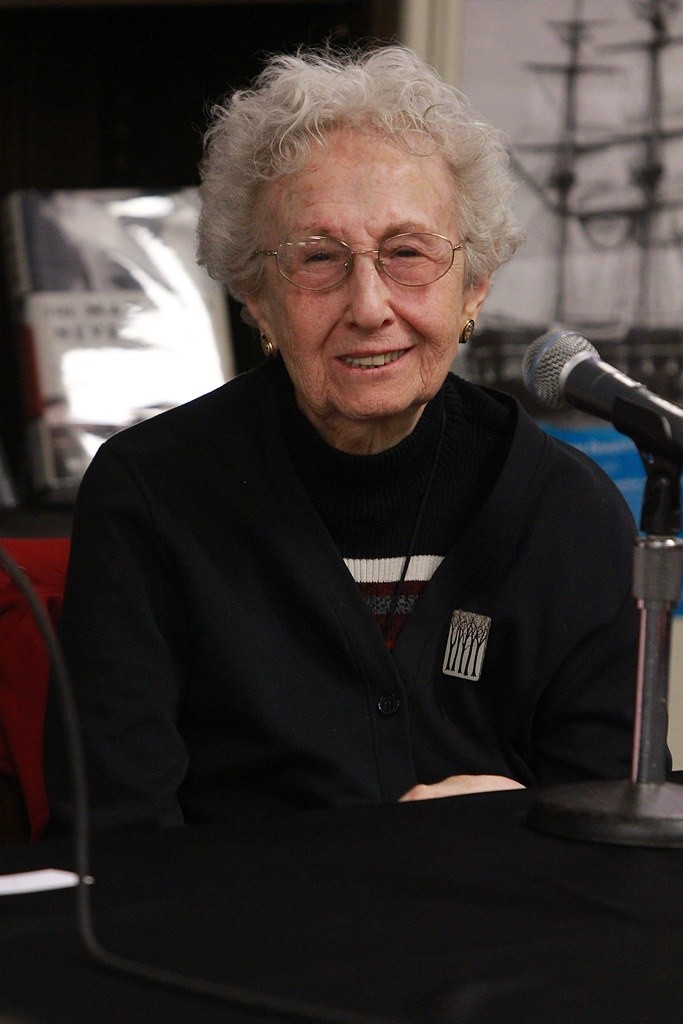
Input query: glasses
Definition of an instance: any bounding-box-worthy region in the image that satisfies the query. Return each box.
[254,231,471,292]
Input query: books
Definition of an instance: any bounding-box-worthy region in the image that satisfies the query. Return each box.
[0,182,238,502]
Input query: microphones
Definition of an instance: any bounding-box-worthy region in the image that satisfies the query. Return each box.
[522,329,683,460]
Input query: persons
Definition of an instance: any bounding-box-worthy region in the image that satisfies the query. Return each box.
[504,341,682,621]
[41,43,638,1024]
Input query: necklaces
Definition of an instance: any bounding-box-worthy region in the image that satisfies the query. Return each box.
[381,407,446,642]
